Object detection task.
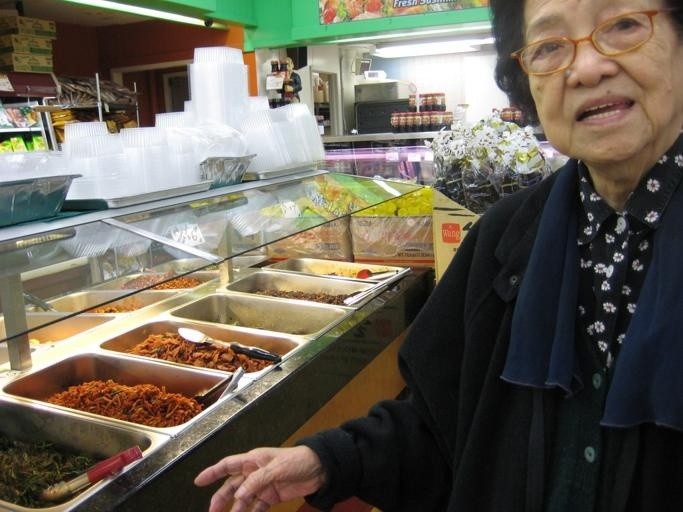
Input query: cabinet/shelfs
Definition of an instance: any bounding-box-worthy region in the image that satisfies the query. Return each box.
[0,71,141,152]
[0,169,433,512]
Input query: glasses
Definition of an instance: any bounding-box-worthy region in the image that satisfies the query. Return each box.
[509,6,679,76]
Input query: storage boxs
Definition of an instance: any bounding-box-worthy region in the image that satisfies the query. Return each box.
[0,9,58,74]
[264,185,480,289]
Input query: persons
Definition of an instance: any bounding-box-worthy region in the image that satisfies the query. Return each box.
[275,56,302,104]
[194,0,683,512]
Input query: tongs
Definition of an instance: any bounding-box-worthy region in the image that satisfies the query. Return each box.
[343,265,414,308]
[192,365,246,400]
[41,444,143,503]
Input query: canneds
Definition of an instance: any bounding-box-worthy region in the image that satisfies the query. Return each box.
[391,93,453,132]
[502,107,523,128]
